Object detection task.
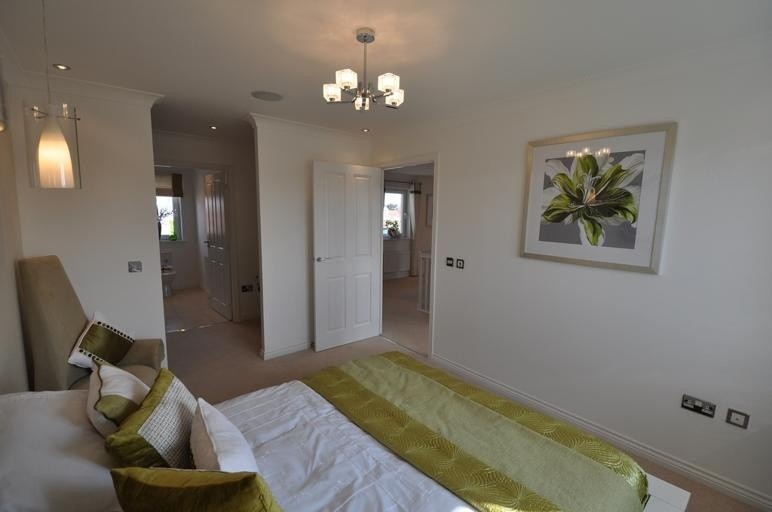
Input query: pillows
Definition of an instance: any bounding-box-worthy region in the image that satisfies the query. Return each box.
[190,398,260,474]
[86,365,151,438]
[110,467,277,510]
[102,368,199,470]
[69,312,136,373]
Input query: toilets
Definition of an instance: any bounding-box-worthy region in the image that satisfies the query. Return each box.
[161,250,176,297]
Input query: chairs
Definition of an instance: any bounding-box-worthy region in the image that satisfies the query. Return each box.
[15,255,165,390]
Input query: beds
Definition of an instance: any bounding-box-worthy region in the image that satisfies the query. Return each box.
[0,350,650,511]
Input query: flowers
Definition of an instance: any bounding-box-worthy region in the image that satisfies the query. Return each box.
[157,207,177,222]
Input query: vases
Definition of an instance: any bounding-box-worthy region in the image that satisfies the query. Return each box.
[158,222,161,239]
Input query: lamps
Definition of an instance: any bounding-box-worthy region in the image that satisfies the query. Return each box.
[321,27,404,112]
[23,1,82,190]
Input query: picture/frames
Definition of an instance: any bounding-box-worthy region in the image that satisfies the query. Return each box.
[518,121,677,276]
